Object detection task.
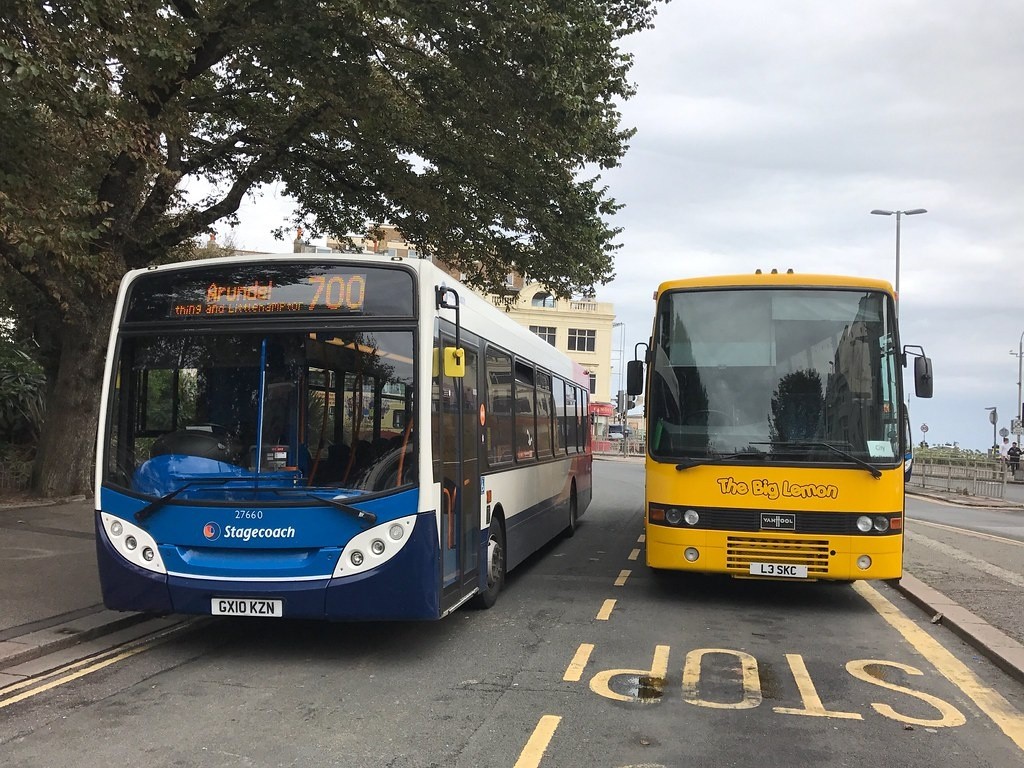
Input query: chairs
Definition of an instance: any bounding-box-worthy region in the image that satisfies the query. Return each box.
[326,436,404,483]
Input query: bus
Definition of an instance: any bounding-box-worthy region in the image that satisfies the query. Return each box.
[609,425,633,439]
[853,398,913,483]
[89,252,593,625]
[628,269,933,582]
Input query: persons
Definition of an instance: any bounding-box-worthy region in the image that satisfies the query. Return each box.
[687,374,758,448]
[999,438,1024,476]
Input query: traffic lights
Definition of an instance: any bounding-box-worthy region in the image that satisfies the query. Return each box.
[628,395,637,410]
[615,390,624,413]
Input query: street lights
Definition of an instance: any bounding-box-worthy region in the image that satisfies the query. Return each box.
[1010,352,1023,447]
[871,208,929,321]
[985,407,997,479]
